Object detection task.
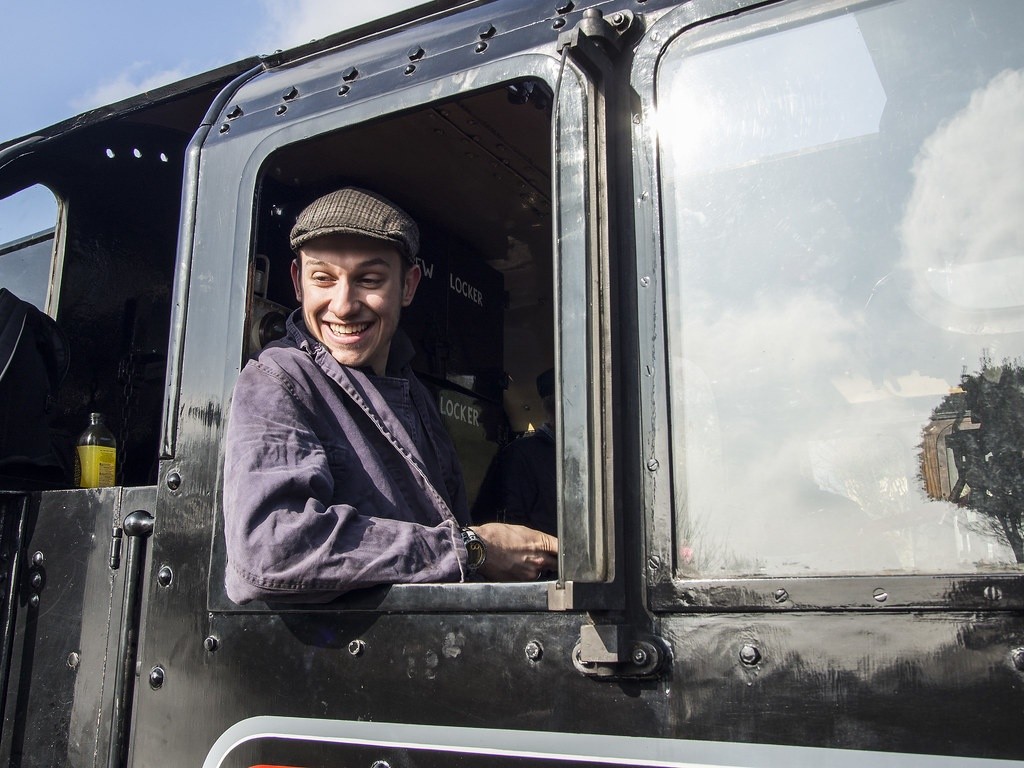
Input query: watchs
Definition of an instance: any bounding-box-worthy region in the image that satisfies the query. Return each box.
[457,527,487,581]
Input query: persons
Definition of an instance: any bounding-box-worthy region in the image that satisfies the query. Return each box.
[223,186,560,606]
[471,367,558,538]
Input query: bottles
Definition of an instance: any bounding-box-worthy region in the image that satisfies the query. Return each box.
[76,412,118,491]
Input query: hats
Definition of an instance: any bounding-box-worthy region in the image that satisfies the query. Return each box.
[535,367,555,399]
[288,184,421,268]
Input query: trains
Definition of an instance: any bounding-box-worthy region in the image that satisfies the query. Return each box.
[0,0,1024,768]
